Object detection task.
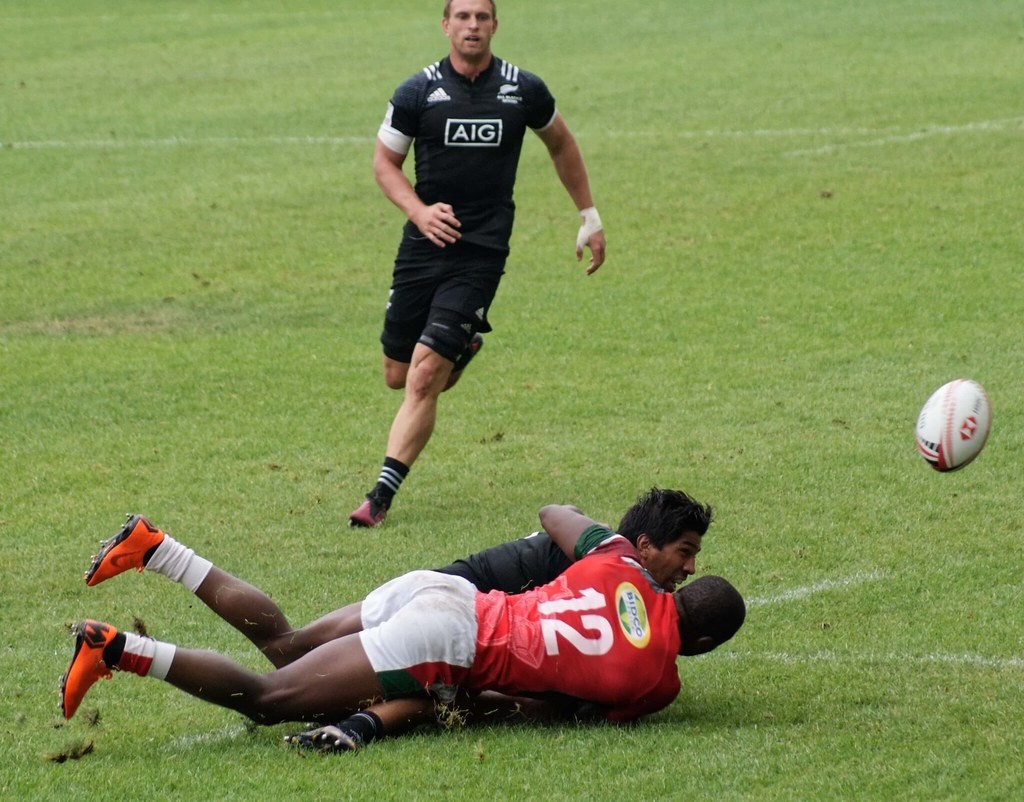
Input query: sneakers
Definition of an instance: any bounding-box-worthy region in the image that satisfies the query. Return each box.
[84,513,164,587]
[58,618,117,719]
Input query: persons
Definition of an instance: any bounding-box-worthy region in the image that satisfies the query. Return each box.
[57,486,748,757]
[348,0,606,529]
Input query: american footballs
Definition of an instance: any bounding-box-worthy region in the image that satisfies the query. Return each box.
[914,376,993,475]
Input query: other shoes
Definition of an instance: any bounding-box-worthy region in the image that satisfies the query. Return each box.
[441,334,483,392]
[349,489,387,525]
[285,724,369,753]
